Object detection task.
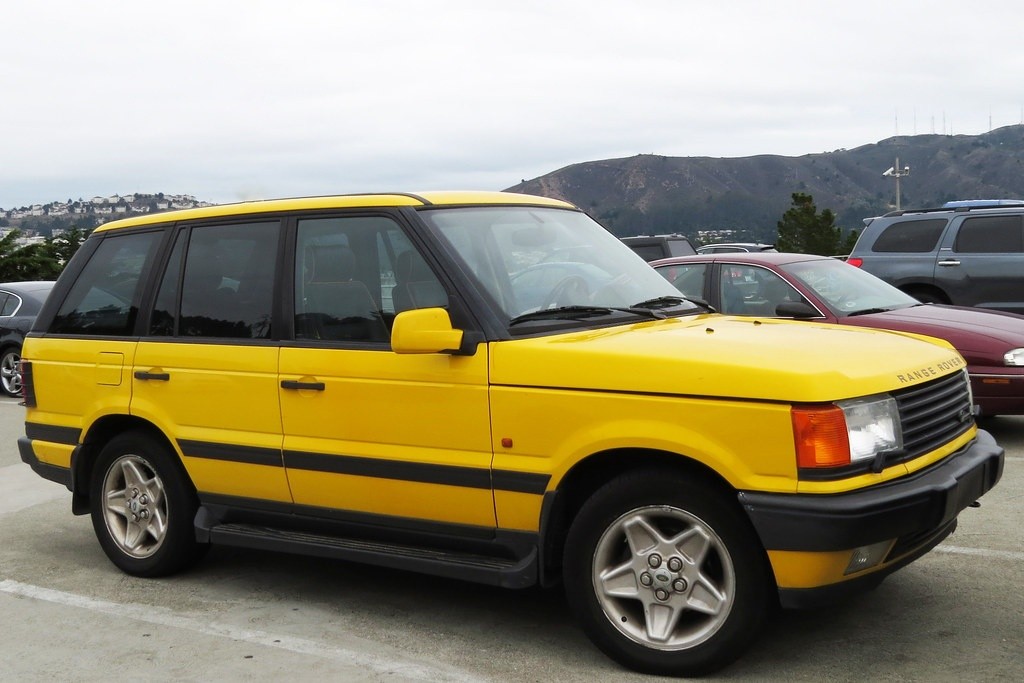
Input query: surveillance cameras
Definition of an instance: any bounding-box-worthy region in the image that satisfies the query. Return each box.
[882,166,910,177]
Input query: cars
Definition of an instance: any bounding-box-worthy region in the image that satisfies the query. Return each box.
[4,281,61,396]
[644,242,1022,425]
[618,231,698,265]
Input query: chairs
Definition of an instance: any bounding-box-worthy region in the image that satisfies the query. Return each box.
[302,245,392,346]
[392,248,454,313]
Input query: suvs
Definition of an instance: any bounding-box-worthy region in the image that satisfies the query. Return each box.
[19,191,1005,677]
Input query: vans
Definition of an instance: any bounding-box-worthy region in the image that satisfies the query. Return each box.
[850,205,1022,317]
